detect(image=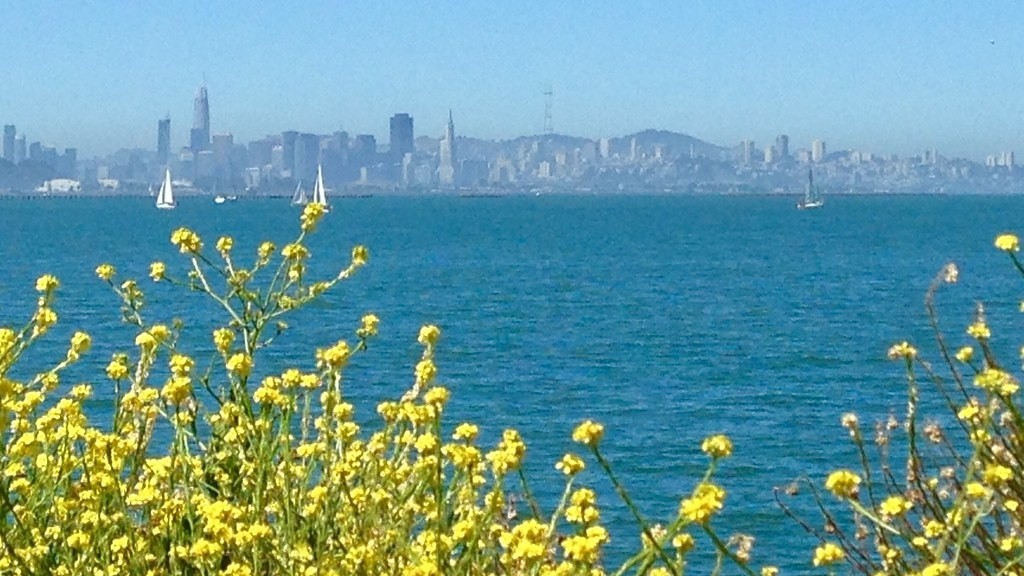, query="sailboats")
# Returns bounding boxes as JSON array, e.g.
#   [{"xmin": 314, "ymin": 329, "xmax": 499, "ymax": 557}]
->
[
  {"xmin": 793, "ymin": 164, "xmax": 824, "ymax": 210},
  {"xmin": 306, "ymin": 164, "xmax": 335, "ymax": 215},
  {"xmin": 289, "ymin": 179, "xmax": 311, "ymax": 208},
  {"xmin": 156, "ymin": 169, "xmax": 178, "ymax": 212}
]
